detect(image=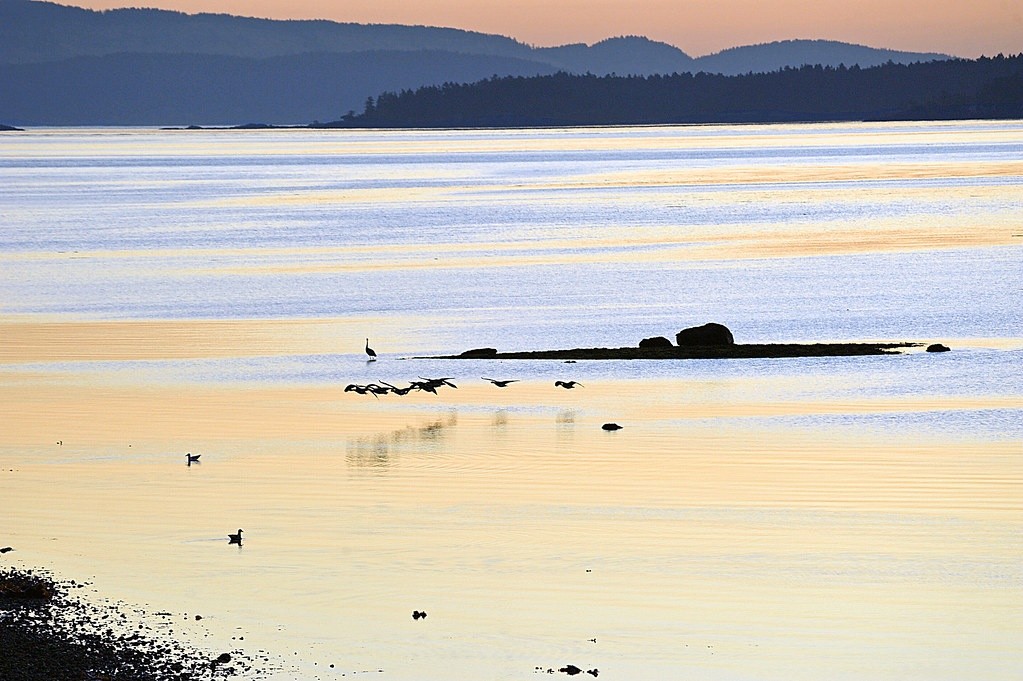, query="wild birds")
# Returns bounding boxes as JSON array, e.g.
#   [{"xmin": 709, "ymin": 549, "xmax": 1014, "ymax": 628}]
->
[{"xmin": 182, "ymin": 336, "xmax": 584, "ymax": 547}]
